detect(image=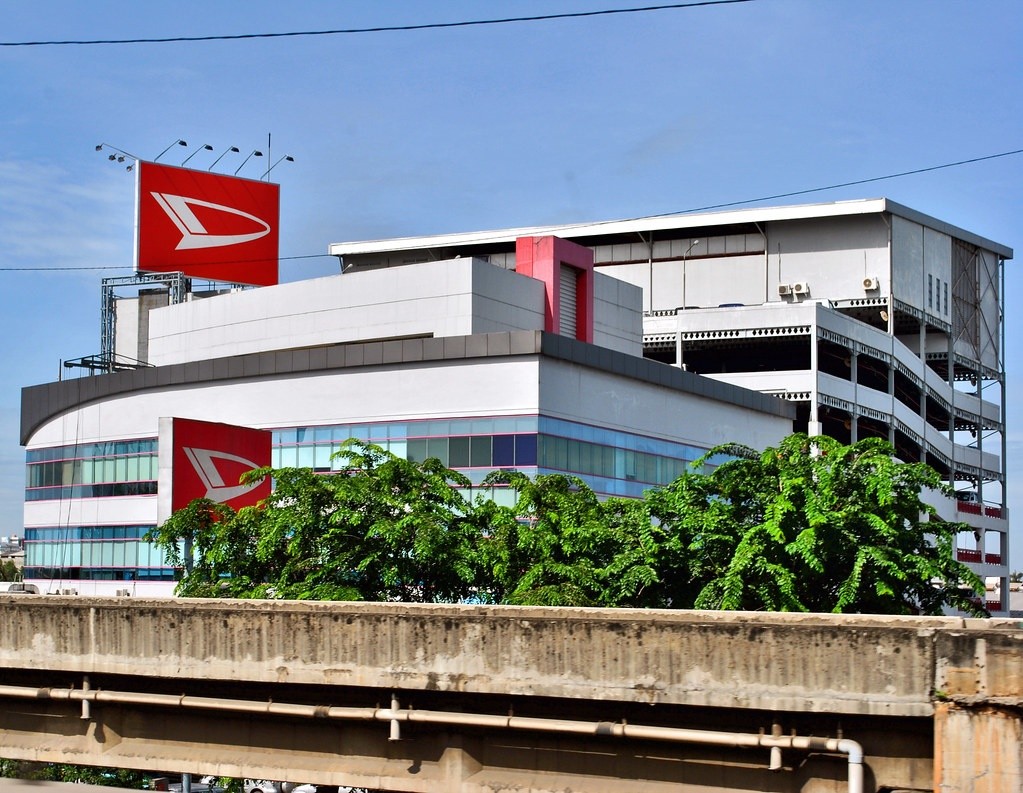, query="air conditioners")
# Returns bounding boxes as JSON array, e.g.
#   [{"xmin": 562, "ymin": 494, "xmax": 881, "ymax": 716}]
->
[
  {"xmin": 779, "ymin": 284, "xmax": 792, "ymax": 296},
  {"xmin": 861, "ymin": 277, "xmax": 878, "ymax": 290},
  {"xmin": 795, "ymin": 283, "xmax": 809, "ymax": 295}
]
